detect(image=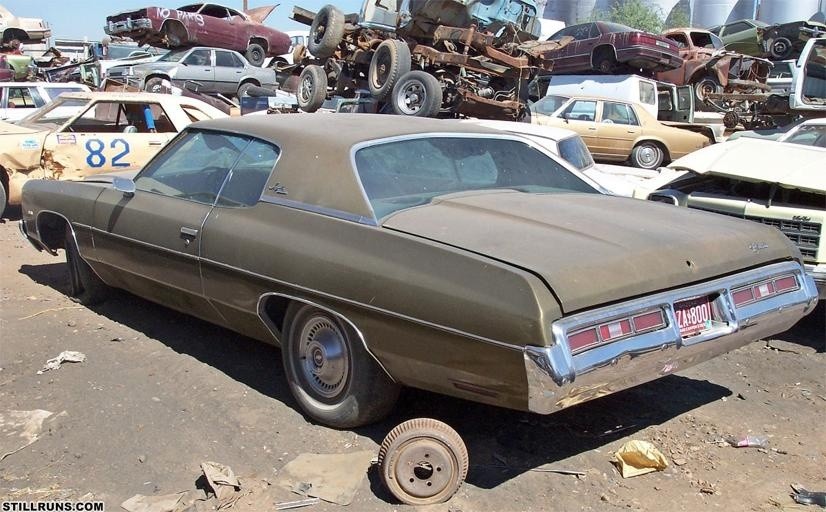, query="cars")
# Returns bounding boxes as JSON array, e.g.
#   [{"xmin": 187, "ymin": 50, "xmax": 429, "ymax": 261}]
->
[{"xmin": 0, "ymin": 0, "xmax": 826, "ymax": 429}]
